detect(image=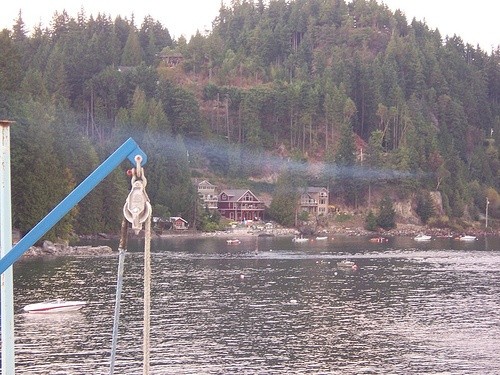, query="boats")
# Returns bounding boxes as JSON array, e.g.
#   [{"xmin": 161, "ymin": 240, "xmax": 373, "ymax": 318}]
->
[
  {"xmin": 316, "ymin": 236, "xmax": 329, "ymax": 240},
  {"xmin": 413, "ymin": 233, "xmax": 432, "ymax": 240},
  {"xmin": 336, "ymin": 260, "xmax": 355, "ymax": 267},
  {"xmin": 23, "ymin": 297, "xmax": 89, "ymax": 313},
  {"xmin": 454, "ymin": 235, "xmax": 477, "ymax": 241},
  {"xmin": 227, "ymin": 239, "xmax": 241, "ymax": 246},
  {"xmin": 292, "ymin": 235, "xmax": 309, "ymax": 242}
]
